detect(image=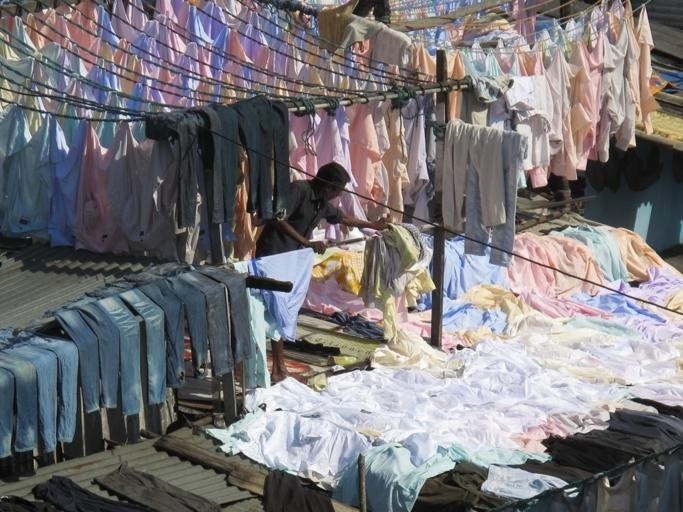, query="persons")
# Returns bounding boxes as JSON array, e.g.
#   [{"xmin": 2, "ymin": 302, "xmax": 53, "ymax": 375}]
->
[{"xmin": 255, "ymin": 162, "xmax": 392, "ymax": 258}]
